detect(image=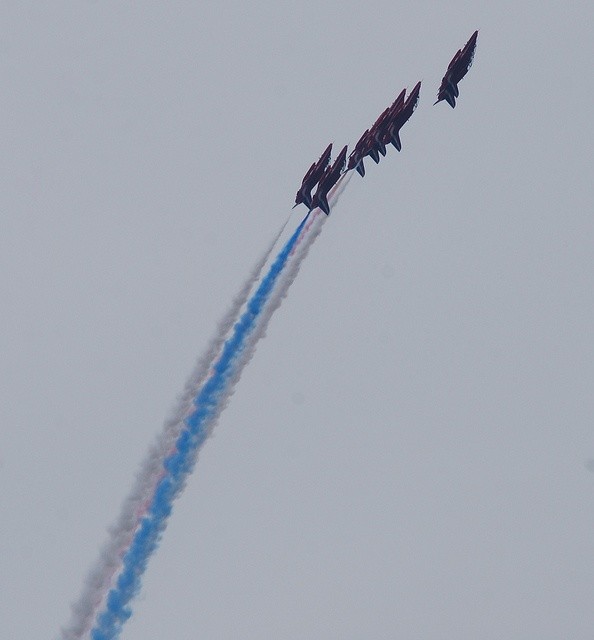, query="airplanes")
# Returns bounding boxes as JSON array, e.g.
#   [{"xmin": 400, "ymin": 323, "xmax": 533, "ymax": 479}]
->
[
  {"xmin": 434, "ymin": 30, "xmax": 478, "ymax": 108},
  {"xmin": 343, "ymin": 80, "xmax": 421, "ymax": 178},
  {"xmin": 293, "ymin": 143, "xmax": 347, "ymax": 214}
]
